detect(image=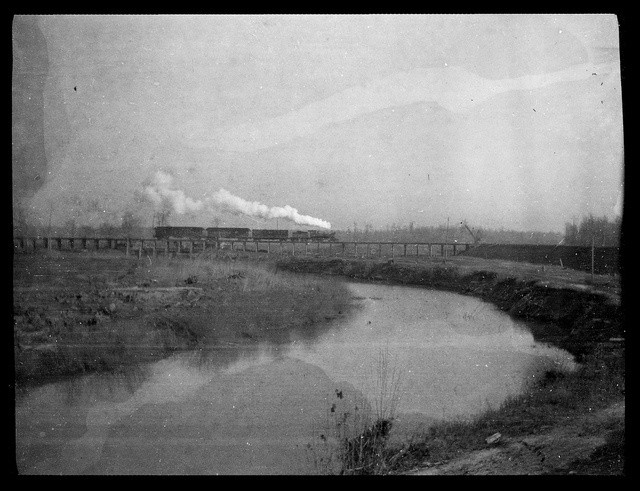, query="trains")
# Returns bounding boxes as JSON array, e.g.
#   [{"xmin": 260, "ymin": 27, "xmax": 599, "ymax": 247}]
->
[{"xmin": 152, "ymin": 226, "xmax": 336, "ymax": 241}]
